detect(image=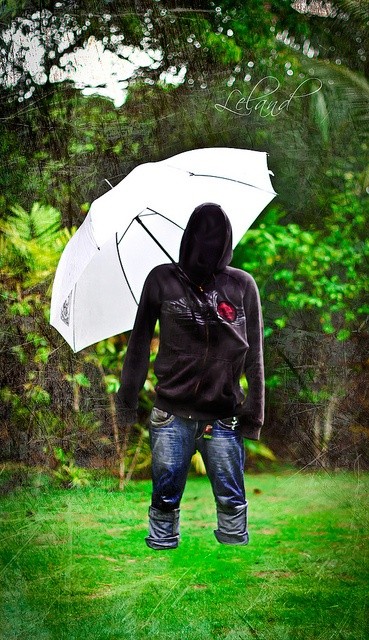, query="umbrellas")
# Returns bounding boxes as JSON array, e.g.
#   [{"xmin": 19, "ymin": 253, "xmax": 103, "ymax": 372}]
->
[{"xmin": 47, "ymin": 144, "xmax": 280, "ymax": 356}]
